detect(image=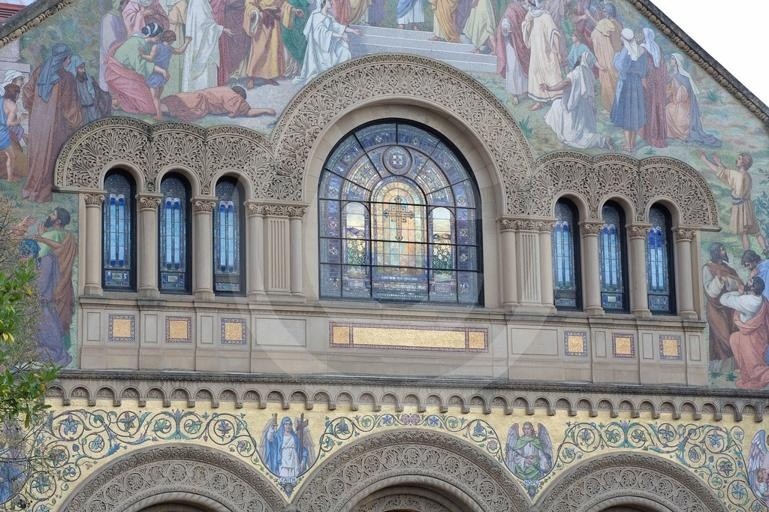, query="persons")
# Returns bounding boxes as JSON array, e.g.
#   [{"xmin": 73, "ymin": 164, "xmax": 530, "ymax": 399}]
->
[
  {"xmin": 511, "ymin": 422, "xmax": 551, "ymax": 482},
  {"xmin": 265, "ymin": 416, "xmax": 309, "ymax": 479}
]
